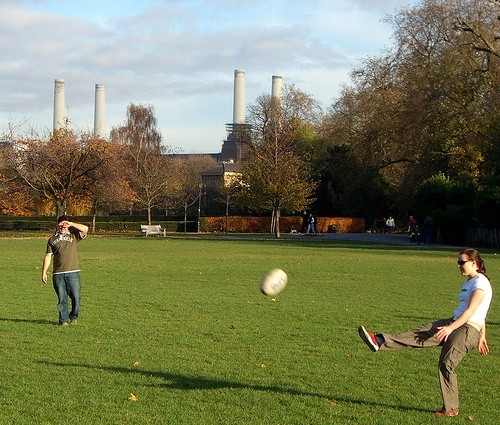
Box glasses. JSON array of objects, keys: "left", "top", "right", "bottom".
[{"left": 458, "top": 261, "right": 470, "bottom": 265}]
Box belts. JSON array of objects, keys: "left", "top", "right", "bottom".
[{"left": 451, "top": 315, "right": 481, "bottom": 333}]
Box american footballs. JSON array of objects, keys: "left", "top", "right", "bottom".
[{"left": 259, "top": 268, "right": 289, "bottom": 297}]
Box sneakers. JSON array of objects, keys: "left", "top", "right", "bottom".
[
  {"left": 358, "top": 325, "right": 380, "bottom": 352},
  {"left": 435, "top": 409, "right": 459, "bottom": 416}
]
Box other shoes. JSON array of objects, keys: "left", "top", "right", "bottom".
[
  {"left": 60, "top": 322, "right": 68, "bottom": 326},
  {"left": 70, "top": 320, "right": 78, "bottom": 325}
]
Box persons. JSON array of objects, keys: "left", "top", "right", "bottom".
[
  {"left": 299, "top": 210, "right": 316, "bottom": 236},
  {"left": 378, "top": 216, "right": 396, "bottom": 235},
  {"left": 41, "top": 215, "right": 89, "bottom": 327},
  {"left": 408, "top": 215, "right": 418, "bottom": 244},
  {"left": 421, "top": 214, "right": 434, "bottom": 245},
  {"left": 359, "top": 249, "right": 492, "bottom": 417}
]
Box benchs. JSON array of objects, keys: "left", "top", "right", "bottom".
[{"left": 138, "top": 224, "right": 167, "bottom": 237}]
